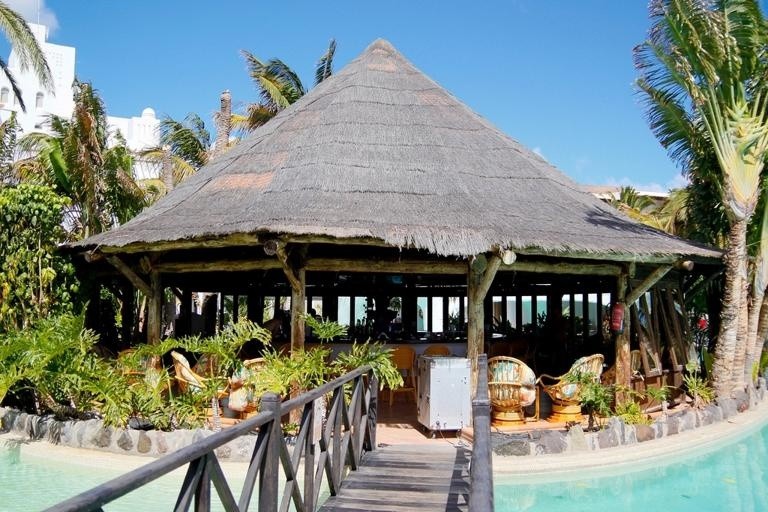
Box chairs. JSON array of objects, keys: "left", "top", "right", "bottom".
[
  {"left": 487, "top": 356, "right": 540, "bottom": 425},
  {"left": 423, "top": 344, "right": 451, "bottom": 356},
  {"left": 380, "top": 344, "right": 417, "bottom": 406},
  {"left": 540, "top": 353, "right": 605, "bottom": 424},
  {"left": 231, "top": 358, "right": 267, "bottom": 419},
  {"left": 118, "top": 350, "right": 167, "bottom": 417},
  {"left": 171, "top": 351, "right": 229, "bottom": 402},
  {"left": 603, "top": 350, "right": 642, "bottom": 383}
]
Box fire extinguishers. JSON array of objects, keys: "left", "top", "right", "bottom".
[{"left": 610, "top": 299, "right": 625, "bottom": 334}]
